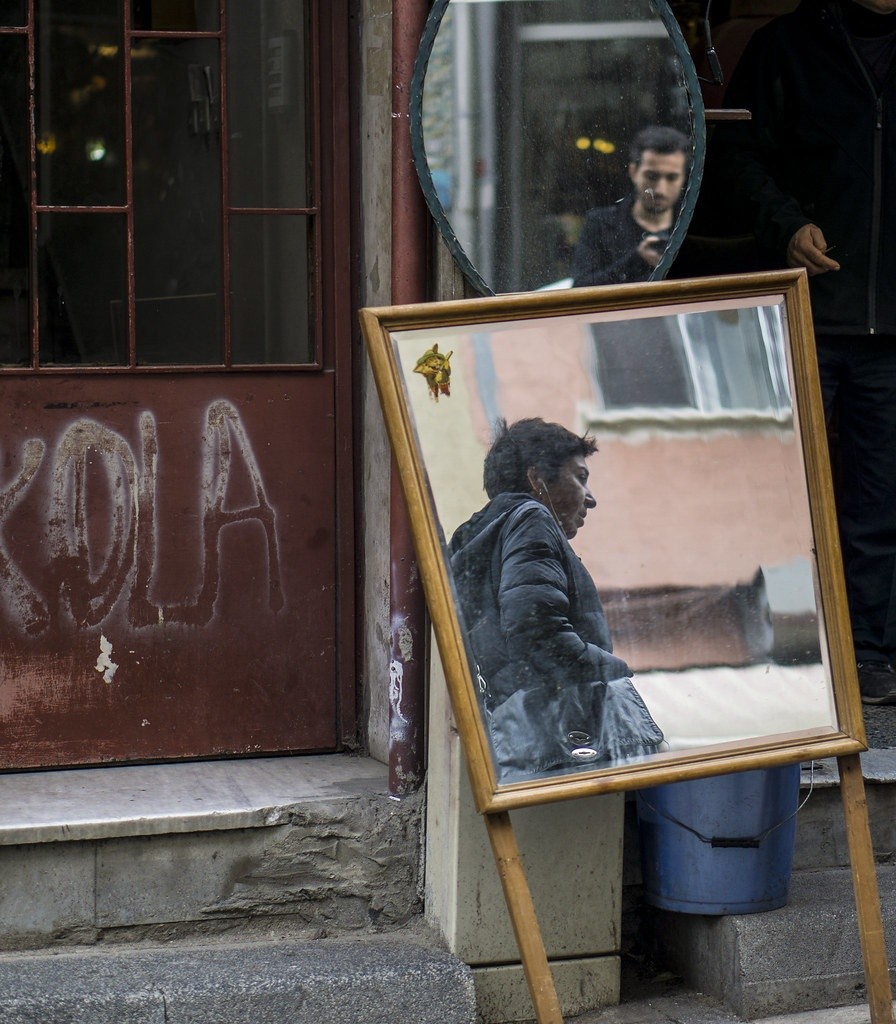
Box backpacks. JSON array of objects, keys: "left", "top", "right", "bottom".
[{"left": 484, "top": 680, "right": 671, "bottom": 783}]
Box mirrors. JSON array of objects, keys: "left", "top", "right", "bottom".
[
  {"left": 409, "top": 0, "right": 706, "bottom": 294},
  {"left": 358, "top": 267, "right": 871, "bottom": 813}
]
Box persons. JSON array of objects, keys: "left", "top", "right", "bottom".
[
  {"left": 708, "top": 0, "right": 896, "bottom": 707},
  {"left": 447, "top": 419, "right": 667, "bottom": 778},
  {"left": 570, "top": 125, "right": 691, "bottom": 288}
]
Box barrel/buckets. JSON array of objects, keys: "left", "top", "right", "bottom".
[{"left": 635, "top": 754, "right": 823, "bottom": 913}]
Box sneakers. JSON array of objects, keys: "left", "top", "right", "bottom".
[{"left": 857, "top": 660, "right": 896, "bottom": 705}]
